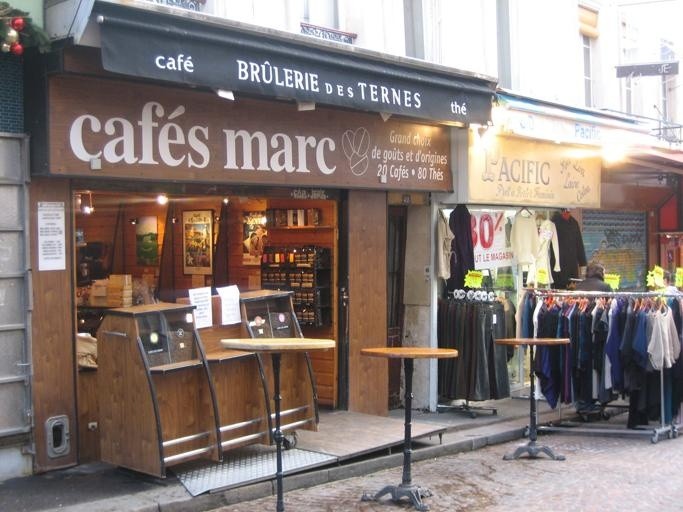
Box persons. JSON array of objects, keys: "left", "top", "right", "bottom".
[
  {"left": 574, "top": 263, "right": 613, "bottom": 292},
  {"left": 657, "top": 272, "right": 683, "bottom": 295}
]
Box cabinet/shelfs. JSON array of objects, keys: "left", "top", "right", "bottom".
[
  {"left": 261, "top": 199, "right": 338, "bottom": 409},
  {"left": 96, "top": 289, "right": 318, "bottom": 479}
]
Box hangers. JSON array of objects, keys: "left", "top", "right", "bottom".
[
  {"left": 515, "top": 207, "right": 532, "bottom": 215},
  {"left": 561, "top": 208, "right": 572, "bottom": 218},
  {"left": 566, "top": 282, "right": 577, "bottom": 288},
  {"left": 536, "top": 212, "right": 547, "bottom": 220},
  {"left": 438, "top": 290, "right": 672, "bottom": 312}
]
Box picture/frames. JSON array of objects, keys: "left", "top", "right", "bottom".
[{"left": 182, "top": 210, "right": 213, "bottom": 274}]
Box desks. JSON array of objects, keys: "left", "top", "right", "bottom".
[
  {"left": 495, "top": 337, "right": 571, "bottom": 460},
  {"left": 219, "top": 338, "right": 336, "bottom": 512},
  {"left": 360, "top": 347, "right": 459, "bottom": 512}
]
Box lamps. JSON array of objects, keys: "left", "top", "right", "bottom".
[
  {"left": 80, "top": 192, "right": 94, "bottom": 216},
  {"left": 168, "top": 216, "right": 176, "bottom": 225},
  {"left": 128, "top": 218, "right": 139, "bottom": 225}
]
